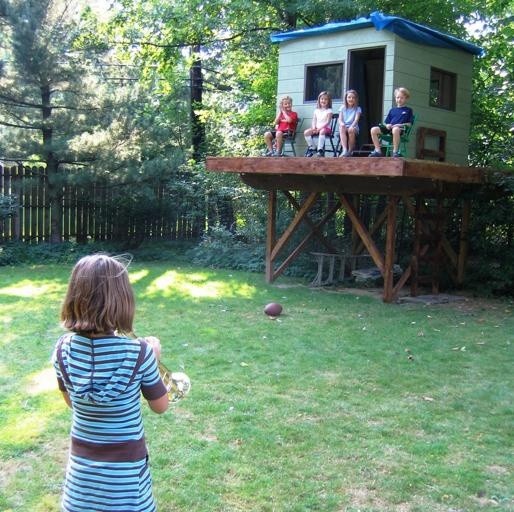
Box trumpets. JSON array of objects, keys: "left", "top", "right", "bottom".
[{"left": 119, "top": 322, "right": 191, "bottom": 405}]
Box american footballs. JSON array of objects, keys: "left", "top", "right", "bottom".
[{"left": 264, "top": 303, "right": 282, "bottom": 315}]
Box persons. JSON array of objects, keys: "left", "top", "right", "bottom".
[
  {"left": 336, "top": 88, "right": 362, "bottom": 157},
  {"left": 258, "top": 94, "right": 298, "bottom": 159},
  {"left": 366, "top": 87, "right": 414, "bottom": 159},
  {"left": 302, "top": 90, "right": 334, "bottom": 158},
  {"left": 49, "top": 250, "right": 173, "bottom": 512}
]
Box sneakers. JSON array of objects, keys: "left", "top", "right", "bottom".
[
  {"left": 264, "top": 151, "right": 282, "bottom": 156},
  {"left": 369, "top": 150, "right": 382, "bottom": 157},
  {"left": 307, "top": 147, "right": 325, "bottom": 157},
  {"left": 339, "top": 150, "right": 351, "bottom": 157},
  {"left": 393, "top": 152, "right": 398, "bottom": 156}
]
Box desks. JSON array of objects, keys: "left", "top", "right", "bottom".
[{"left": 307, "top": 250, "right": 373, "bottom": 289}]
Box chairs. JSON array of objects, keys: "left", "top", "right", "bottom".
[{"left": 264, "top": 112, "right": 421, "bottom": 159}]
